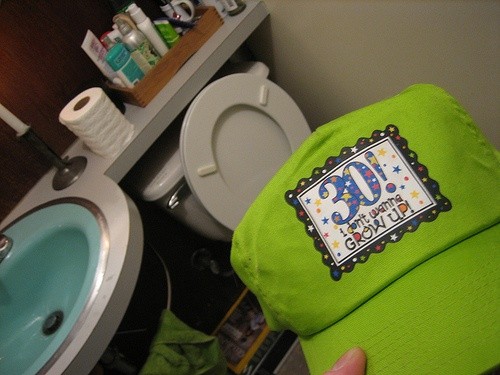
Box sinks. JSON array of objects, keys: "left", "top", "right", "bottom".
[{"left": 0, "top": 196, "right": 111, "bottom": 374}]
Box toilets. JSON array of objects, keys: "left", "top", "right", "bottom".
[{"left": 130, "top": 56, "right": 318, "bottom": 243}]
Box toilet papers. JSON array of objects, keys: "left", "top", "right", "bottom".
[{"left": 57, "top": 85, "right": 134, "bottom": 158}]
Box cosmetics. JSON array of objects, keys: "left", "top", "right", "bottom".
[
  {"left": 81, "top": 29, "right": 123, "bottom": 85},
  {"left": 105, "top": 42, "right": 143, "bottom": 88},
  {"left": 98, "top": 0, "right": 182, "bottom": 76}
]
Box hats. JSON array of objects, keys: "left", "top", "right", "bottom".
[{"left": 230, "top": 83, "right": 499, "bottom": 375}]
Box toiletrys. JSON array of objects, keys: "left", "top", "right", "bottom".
[{"left": 70, "top": 0, "right": 200, "bottom": 85}]
